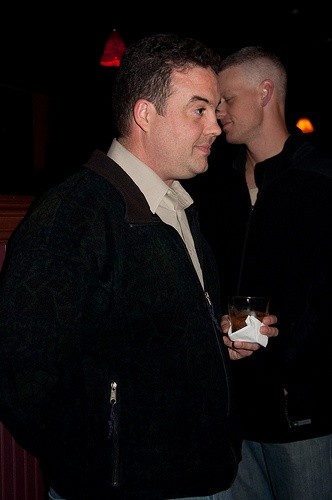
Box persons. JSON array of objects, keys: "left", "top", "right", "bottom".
[
  {"left": 1, "top": 33, "right": 279, "bottom": 500},
  {"left": 209, "top": 47, "right": 332, "bottom": 500}
]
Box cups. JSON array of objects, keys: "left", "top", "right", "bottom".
[{"left": 229, "top": 295, "right": 271, "bottom": 333}]
